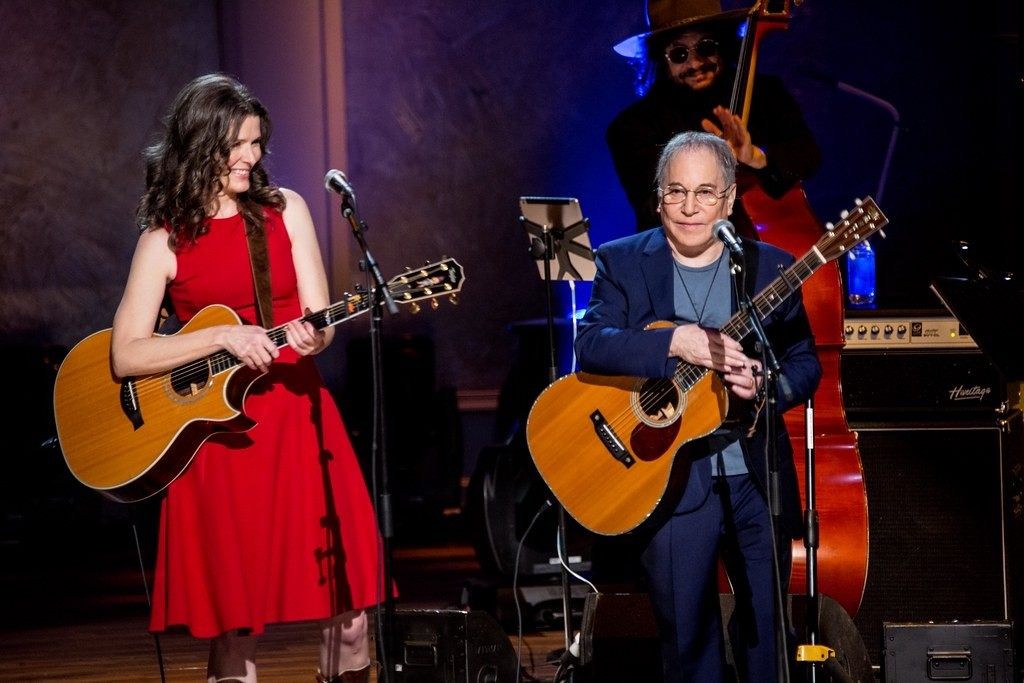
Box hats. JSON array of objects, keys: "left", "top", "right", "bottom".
[{"left": 645, "top": 0, "right": 751, "bottom": 43}]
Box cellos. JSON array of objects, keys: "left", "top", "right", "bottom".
[{"left": 712, "top": 0, "right": 873, "bottom": 634}]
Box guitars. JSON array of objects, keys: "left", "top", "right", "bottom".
[
  {"left": 523, "top": 192, "right": 893, "bottom": 547},
  {"left": 52, "top": 253, "right": 467, "bottom": 506}
]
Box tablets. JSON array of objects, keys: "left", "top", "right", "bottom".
[{"left": 520, "top": 195, "right": 596, "bottom": 281}]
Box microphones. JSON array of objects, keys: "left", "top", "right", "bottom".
[
  {"left": 324, "top": 168, "right": 356, "bottom": 198},
  {"left": 711, "top": 219, "right": 745, "bottom": 257}
]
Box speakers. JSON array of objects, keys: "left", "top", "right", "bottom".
[
  {"left": 578, "top": 592, "right": 877, "bottom": 682},
  {"left": 372, "top": 607, "right": 529, "bottom": 683},
  {"left": 846, "top": 418, "right": 1011, "bottom": 675}
]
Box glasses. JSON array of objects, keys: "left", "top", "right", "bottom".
[
  {"left": 663, "top": 185, "right": 730, "bottom": 206},
  {"left": 665, "top": 38, "right": 720, "bottom": 64}
]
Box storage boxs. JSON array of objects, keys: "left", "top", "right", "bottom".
[{"left": 883, "top": 620, "right": 1024, "bottom": 683}]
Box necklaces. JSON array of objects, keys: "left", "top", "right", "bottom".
[{"left": 674, "top": 245, "right": 726, "bottom": 325}]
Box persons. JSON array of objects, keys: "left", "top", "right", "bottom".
[
  {"left": 606, "top": 0, "right": 824, "bottom": 231},
  {"left": 111, "top": 74, "right": 398, "bottom": 683},
  {"left": 572, "top": 131, "right": 824, "bottom": 683}
]
